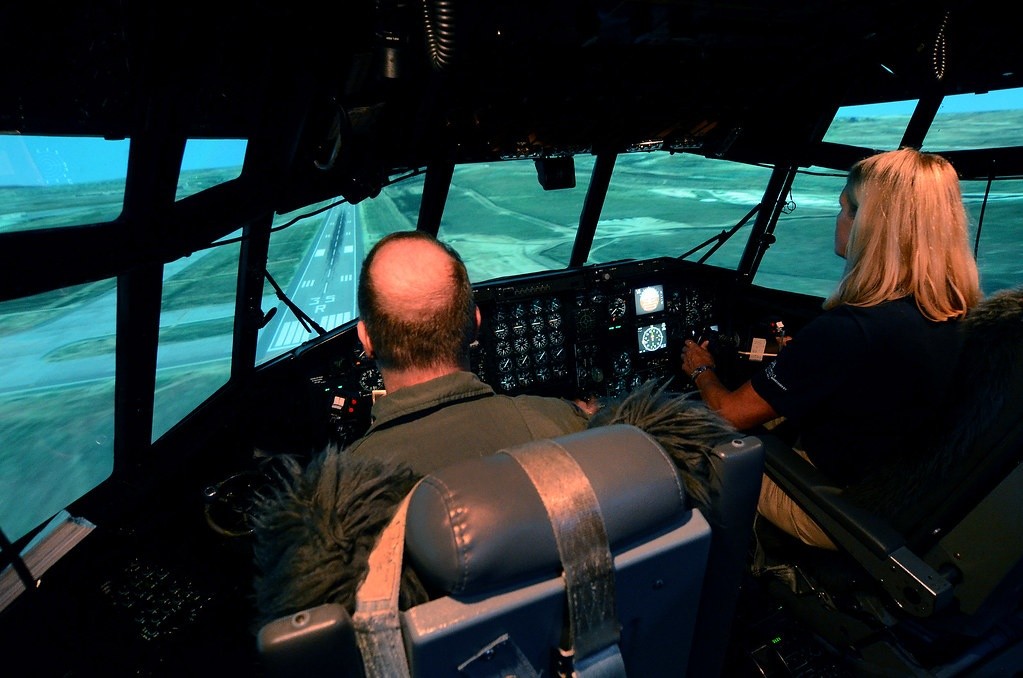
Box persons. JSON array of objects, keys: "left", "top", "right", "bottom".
[
  {"left": 240, "top": 232, "right": 591, "bottom": 639},
  {"left": 680, "top": 148, "right": 983, "bottom": 549}
]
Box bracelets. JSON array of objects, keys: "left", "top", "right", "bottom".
[{"left": 691, "top": 365, "right": 713, "bottom": 381}]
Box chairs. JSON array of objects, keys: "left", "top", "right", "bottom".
[
  {"left": 740, "top": 423, "right": 1023, "bottom": 678},
  {"left": 257, "top": 421, "right": 765, "bottom": 678}
]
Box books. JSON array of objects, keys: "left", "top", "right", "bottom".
[{"left": 0, "top": 509, "right": 97, "bottom": 615}]
